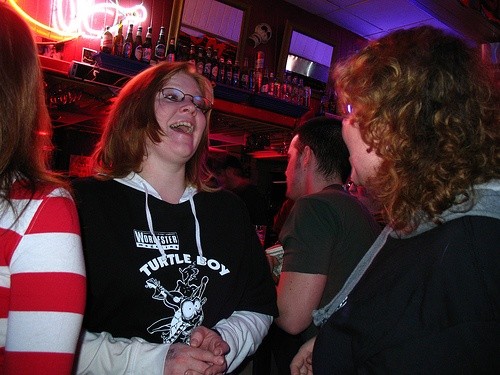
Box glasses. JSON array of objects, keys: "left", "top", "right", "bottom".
[{"left": 159, "top": 87, "right": 215, "bottom": 114}]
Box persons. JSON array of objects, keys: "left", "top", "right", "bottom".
[
  {"left": 71, "top": 61, "right": 280, "bottom": 375},
  {"left": 0, "top": 3, "right": 87, "bottom": 375},
  {"left": 213, "top": 117, "right": 382, "bottom": 375},
  {"left": 290, "top": 25, "right": 500, "bottom": 375}
]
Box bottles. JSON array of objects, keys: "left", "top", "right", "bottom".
[
  {"left": 233, "top": 55, "right": 241, "bottom": 88},
  {"left": 178, "top": 46, "right": 187, "bottom": 63},
  {"left": 113, "top": 19, "right": 124, "bottom": 57},
  {"left": 134, "top": 26, "right": 143, "bottom": 62},
  {"left": 218, "top": 53, "right": 226, "bottom": 84},
  {"left": 250, "top": 65, "right": 312, "bottom": 110},
  {"left": 166, "top": 37, "right": 177, "bottom": 62},
  {"left": 226, "top": 55, "right": 233, "bottom": 86},
  {"left": 196, "top": 46, "right": 204, "bottom": 75},
  {"left": 241, "top": 57, "right": 249, "bottom": 89},
  {"left": 124, "top": 24, "right": 134, "bottom": 59},
  {"left": 204, "top": 47, "right": 212, "bottom": 80},
  {"left": 188, "top": 45, "right": 196, "bottom": 72},
  {"left": 211, "top": 50, "right": 219, "bottom": 83},
  {"left": 142, "top": 26, "right": 153, "bottom": 64},
  {"left": 100, "top": 26, "right": 113, "bottom": 54},
  {"left": 153, "top": 26, "right": 166, "bottom": 64}
]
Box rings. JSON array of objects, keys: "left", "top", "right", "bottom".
[{"left": 184, "top": 369, "right": 190, "bottom": 375}]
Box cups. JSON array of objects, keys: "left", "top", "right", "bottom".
[{"left": 253, "top": 224, "right": 266, "bottom": 247}]
